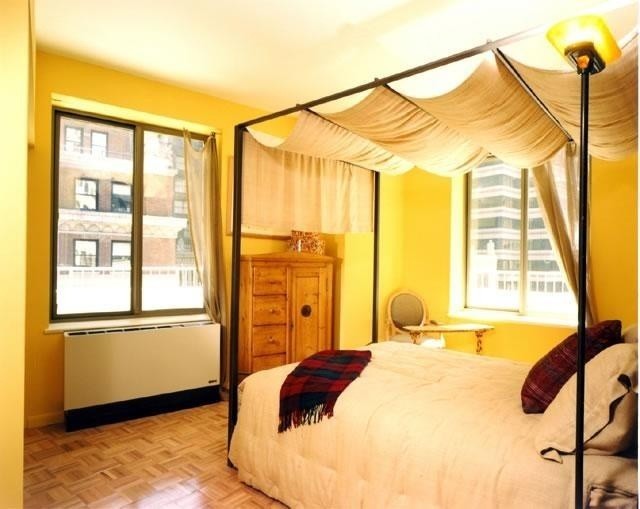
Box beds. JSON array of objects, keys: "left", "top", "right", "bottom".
[{"left": 226, "top": 0, "right": 638, "bottom": 509}]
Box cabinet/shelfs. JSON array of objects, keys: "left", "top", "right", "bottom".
[{"left": 238, "top": 256, "right": 334, "bottom": 384}]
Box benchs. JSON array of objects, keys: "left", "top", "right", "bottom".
[{"left": 402, "top": 323, "right": 495, "bottom": 356}]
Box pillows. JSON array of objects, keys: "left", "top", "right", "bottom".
[
  {"left": 521, "top": 319, "right": 622, "bottom": 415},
  {"left": 533, "top": 343, "right": 638, "bottom": 464},
  {"left": 621, "top": 325, "right": 638, "bottom": 343}
]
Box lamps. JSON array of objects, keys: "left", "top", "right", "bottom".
[{"left": 546, "top": 14, "right": 622, "bottom": 509}]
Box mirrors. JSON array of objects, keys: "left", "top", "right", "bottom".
[{"left": 386, "top": 291, "right": 426, "bottom": 334}]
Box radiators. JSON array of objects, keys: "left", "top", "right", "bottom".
[{"left": 64, "top": 321, "right": 221, "bottom": 433}]
